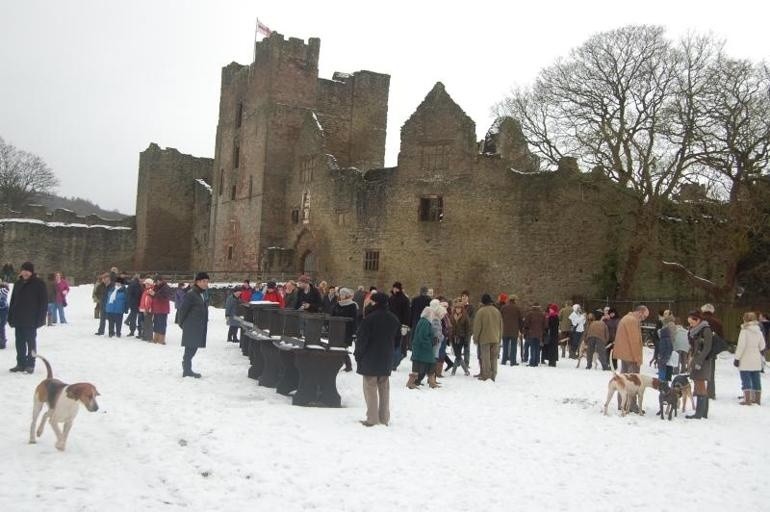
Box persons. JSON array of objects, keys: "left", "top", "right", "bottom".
[
  {"left": 225, "top": 275, "right": 622, "bottom": 389},
  {"left": 174, "top": 271, "right": 210, "bottom": 378},
  {"left": 612, "top": 303, "right": 725, "bottom": 419},
  {"left": 354, "top": 292, "right": 402, "bottom": 427},
  {"left": 93, "top": 267, "right": 172, "bottom": 345},
  {"left": 1, "top": 262, "right": 70, "bottom": 375},
  {"left": 176, "top": 282, "right": 210, "bottom": 322},
  {"left": 734, "top": 312, "right": 770, "bottom": 406}
]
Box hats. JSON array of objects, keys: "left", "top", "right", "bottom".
[
  {"left": 195, "top": 272, "right": 210, "bottom": 280},
  {"left": 98, "top": 268, "right": 164, "bottom": 286},
  {"left": 701, "top": 304, "right": 715, "bottom": 314},
  {"left": 608, "top": 309, "right": 616, "bottom": 314},
  {"left": 548, "top": 304, "right": 559, "bottom": 312},
  {"left": 663, "top": 310, "right": 675, "bottom": 322},
  {"left": 232, "top": 277, "right": 519, "bottom": 323},
  {"left": 20, "top": 262, "right": 36, "bottom": 273},
  {"left": 532, "top": 302, "right": 541, "bottom": 308}
]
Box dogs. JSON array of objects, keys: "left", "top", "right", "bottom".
[
  {"left": 670, "top": 373, "right": 696, "bottom": 414},
  {"left": 603, "top": 348, "right": 661, "bottom": 417},
  {"left": 28, "top": 352, "right": 101, "bottom": 451},
  {"left": 658, "top": 385, "right": 679, "bottom": 420}
]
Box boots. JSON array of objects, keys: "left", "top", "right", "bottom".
[
  {"left": 737, "top": 388, "right": 761, "bottom": 405},
  {"left": 685, "top": 395, "right": 710, "bottom": 419}
]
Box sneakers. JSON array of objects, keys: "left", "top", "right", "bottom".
[
  {"left": 93, "top": 313, "right": 166, "bottom": 344},
  {"left": 0, "top": 318, "right": 68, "bottom": 349},
  {"left": 9, "top": 365, "right": 35, "bottom": 374},
  {"left": 404, "top": 351, "right": 690, "bottom": 389},
  {"left": 360, "top": 419, "right": 389, "bottom": 427},
  {"left": 227, "top": 337, "right": 241, "bottom": 343},
  {"left": 183, "top": 371, "right": 202, "bottom": 378}
]
